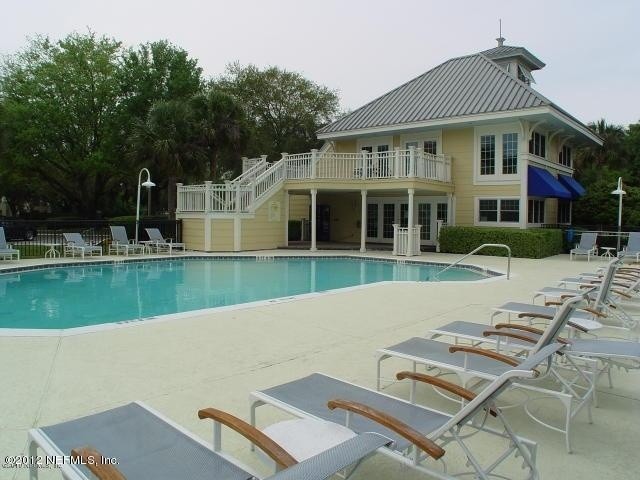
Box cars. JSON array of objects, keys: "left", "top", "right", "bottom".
[{"left": 0, "top": 218, "right": 38, "bottom": 243}]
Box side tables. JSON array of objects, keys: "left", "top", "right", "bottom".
[
  {"left": 254, "top": 417, "right": 358, "bottom": 480},
  {"left": 138, "top": 241, "right": 154, "bottom": 255},
  {"left": 41, "top": 244, "right": 63, "bottom": 260}
]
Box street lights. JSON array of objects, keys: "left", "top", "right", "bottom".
[
  {"left": 610, "top": 176, "right": 629, "bottom": 260},
  {"left": 135, "top": 166, "right": 157, "bottom": 254}
]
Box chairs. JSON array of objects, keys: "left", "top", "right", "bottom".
[
  {"left": 108, "top": 225, "right": 145, "bottom": 257},
  {"left": 26, "top": 399, "right": 395, "bottom": 480},
  {"left": 62, "top": 232, "right": 102, "bottom": 260},
  {"left": 143, "top": 227, "right": 186, "bottom": 254},
  {"left": 249, "top": 341, "right": 566, "bottom": 480},
  {"left": 0, "top": 226, "right": 20, "bottom": 264},
  {"left": 375, "top": 231, "right": 640, "bottom": 455}
]
